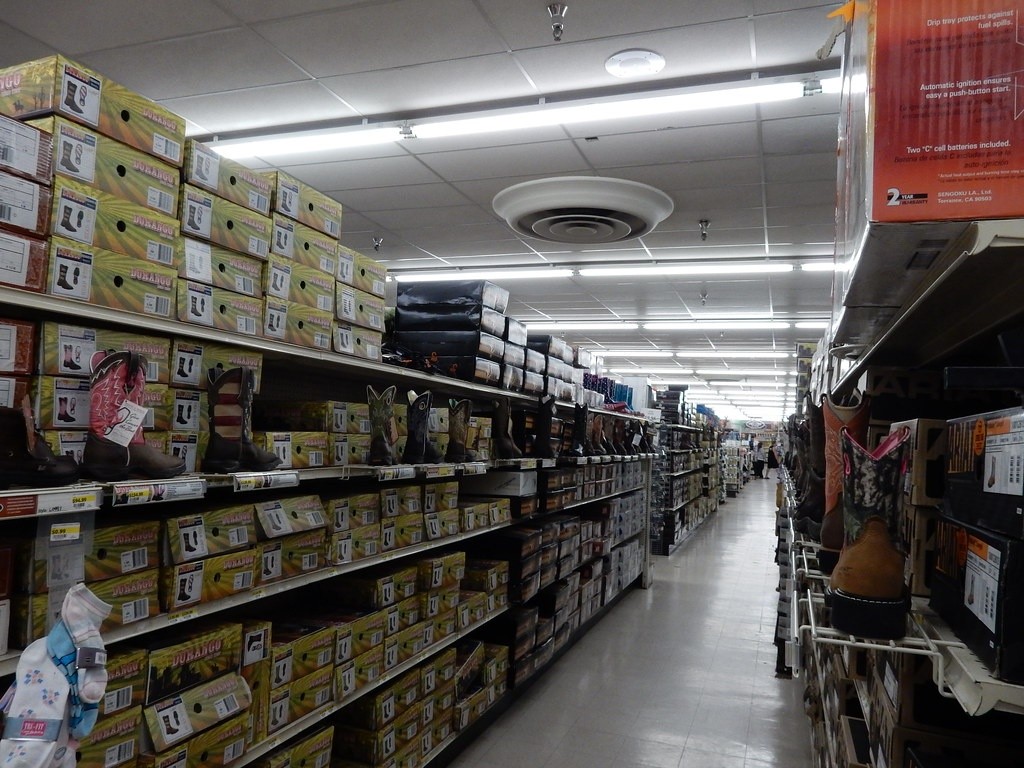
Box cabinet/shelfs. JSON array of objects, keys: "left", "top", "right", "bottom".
[
  {"left": 0, "top": 286, "right": 655, "bottom": 768},
  {"left": 720, "top": 425, "right": 783, "bottom": 498},
  {"left": 655, "top": 423, "right": 728, "bottom": 556},
  {"left": 782, "top": 218, "right": 1024, "bottom": 768}
]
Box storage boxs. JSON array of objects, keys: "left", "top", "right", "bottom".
[
  {"left": 871, "top": 698, "right": 1024, "bottom": 768},
  {"left": 794, "top": 1, "right": 1024, "bottom": 418},
  {"left": 460, "top": 462, "right": 645, "bottom": 517},
  {"left": 867, "top": 648, "right": 878, "bottom": 700},
  {"left": 929, "top": 512, "right": 1024, "bottom": 687},
  {"left": 839, "top": 638, "right": 867, "bottom": 680},
  {"left": 866, "top": 426, "right": 888, "bottom": 450},
  {"left": 663, "top": 427, "right": 721, "bottom": 544},
  {"left": 381, "top": 280, "right": 634, "bottom": 411},
  {"left": 945, "top": 405, "right": 1024, "bottom": 540},
  {"left": 876, "top": 643, "right": 1024, "bottom": 751},
  {"left": 836, "top": 714, "right": 871, "bottom": 768},
  {"left": 889, "top": 419, "right": 949, "bottom": 506},
  {"left": 896, "top": 504, "right": 944, "bottom": 597},
  {"left": 826, "top": 657, "right": 865, "bottom": 731},
  {"left": 622, "top": 376, "right": 708, "bottom": 428},
  {"left": 512, "top": 538, "right": 644, "bottom": 686},
  {"left": 0, "top": 55, "right": 387, "bottom": 363},
  {"left": 512, "top": 490, "right": 645, "bottom": 604},
  {"left": 0, "top": 482, "right": 511, "bottom": 656},
  {"left": 1, "top": 319, "right": 493, "bottom": 469},
  {"left": 833, "top": 653, "right": 866, "bottom": 734},
  {"left": 513, "top": 412, "right": 575, "bottom": 454},
  {"left": 867, "top": 367, "right": 946, "bottom": 425},
  {"left": 75, "top": 551, "right": 509, "bottom": 768},
  {"left": 816, "top": 583, "right": 832, "bottom": 627}
]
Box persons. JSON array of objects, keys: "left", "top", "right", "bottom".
[
  {"left": 749, "top": 436, "right": 765, "bottom": 479},
  {"left": 764, "top": 439, "right": 782, "bottom": 480}
]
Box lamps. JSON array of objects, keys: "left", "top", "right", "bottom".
[{"left": 210, "top": 77, "right": 843, "bottom": 159}]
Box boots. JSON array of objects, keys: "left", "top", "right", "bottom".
[
  {"left": 818, "top": 388, "right": 872, "bottom": 572},
  {"left": 524, "top": 394, "right": 556, "bottom": 459},
  {"left": 680, "top": 432, "right": 697, "bottom": 450},
  {"left": 489, "top": 397, "right": 523, "bottom": 460},
  {"left": 78, "top": 347, "right": 188, "bottom": 482},
  {"left": 784, "top": 387, "right": 826, "bottom": 542},
  {"left": 709, "top": 425, "right": 716, "bottom": 441},
  {"left": 823, "top": 423, "right": 914, "bottom": 641},
  {"left": 716, "top": 432, "right": 723, "bottom": 448},
  {"left": 559, "top": 401, "right": 656, "bottom": 457},
  {"left": 400, "top": 389, "right": 445, "bottom": 464},
  {"left": 444, "top": 398, "right": 488, "bottom": 464},
  {"left": 200, "top": 361, "right": 284, "bottom": 474},
  {"left": 366, "top": 385, "right": 402, "bottom": 467},
  {"left": 702, "top": 422, "right": 710, "bottom": 441}
]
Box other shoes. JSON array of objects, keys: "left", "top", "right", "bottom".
[
  {"left": 759, "top": 475, "right": 763, "bottom": 479},
  {"left": 776, "top": 476, "right": 781, "bottom": 479},
  {"left": 764, "top": 477, "right": 770, "bottom": 479}
]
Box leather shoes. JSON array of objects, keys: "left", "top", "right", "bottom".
[{"left": 0, "top": 405, "right": 80, "bottom": 491}]
